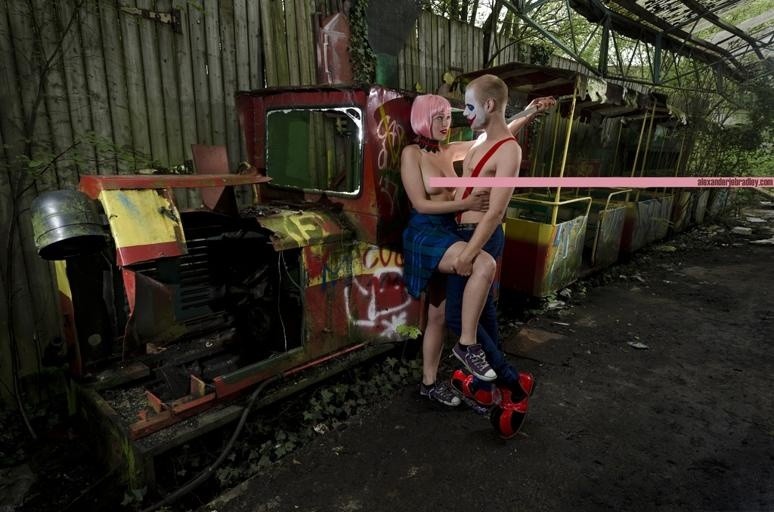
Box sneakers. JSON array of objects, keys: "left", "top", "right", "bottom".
[
  {"left": 451, "top": 341, "right": 497, "bottom": 382},
  {"left": 489, "top": 370, "right": 537, "bottom": 440},
  {"left": 419, "top": 380, "right": 461, "bottom": 407},
  {"left": 450, "top": 367, "right": 496, "bottom": 408}
]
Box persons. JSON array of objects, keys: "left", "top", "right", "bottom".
[
  {"left": 445, "top": 72, "right": 537, "bottom": 440},
  {"left": 400, "top": 94, "right": 556, "bottom": 406}
]
